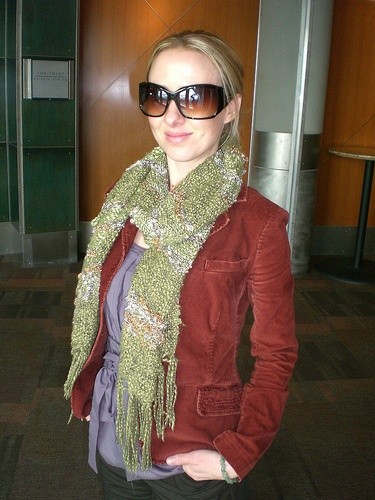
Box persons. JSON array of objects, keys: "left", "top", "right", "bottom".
[{"left": 58, "top": 29, "right": 298, "bottom": 499}]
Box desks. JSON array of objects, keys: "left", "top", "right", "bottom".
[{"left": 313, "top": 144, "right": 375, "bottom": 285}]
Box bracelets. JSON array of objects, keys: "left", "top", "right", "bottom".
[{"left": 220, "top": 453, "right": 240, "bottom": 485}]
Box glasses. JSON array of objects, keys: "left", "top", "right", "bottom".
[{"left": 136, "top": 80, "right": 234, "bottom": 119}]
